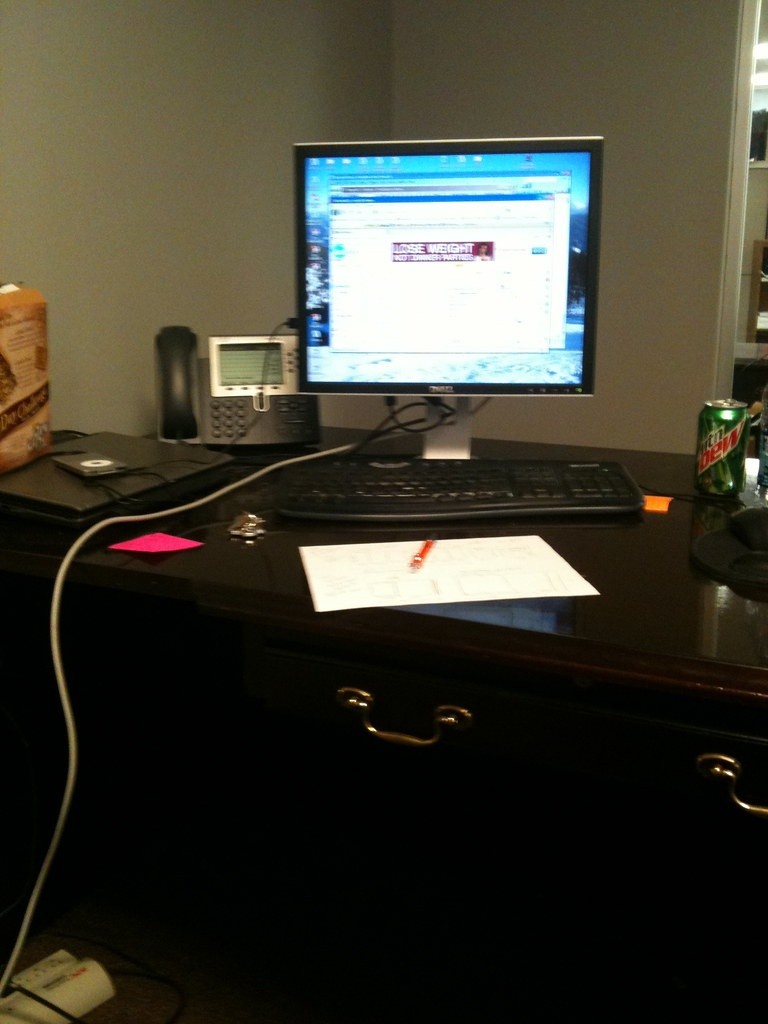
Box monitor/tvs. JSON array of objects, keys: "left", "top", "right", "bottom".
[{"left": 293, "top": 136, "right": 605, "bottom": 460}]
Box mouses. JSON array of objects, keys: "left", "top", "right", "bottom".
[{"left": 727, "top": 507, "right": 768, "bottom": 551}]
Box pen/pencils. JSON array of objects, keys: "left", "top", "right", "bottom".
[{"left": 409, "top": 539, "right": 433, "bottom": 569}]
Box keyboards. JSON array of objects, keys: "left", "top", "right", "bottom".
[{"left": 270, "top": 459, "right": 644, "bottom": 523}]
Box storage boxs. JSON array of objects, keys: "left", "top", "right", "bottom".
[{"left": 0, "top": 280, "right": 51, "bottom": 475}]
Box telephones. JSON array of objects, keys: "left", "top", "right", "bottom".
[{"left": 155, "top": 326, "right": 320, "bottom": 445}]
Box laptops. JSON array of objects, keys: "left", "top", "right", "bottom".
[{"left": 0, "top": 431, "right": 235, "bottom": 529}]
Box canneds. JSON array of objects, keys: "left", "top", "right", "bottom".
[{"left": 694, "top": 398, "right": 751, "bottom": 496}]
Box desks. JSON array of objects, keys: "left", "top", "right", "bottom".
[{"left": 0, "top": 427, "right": 768, "bottom": 957}]
